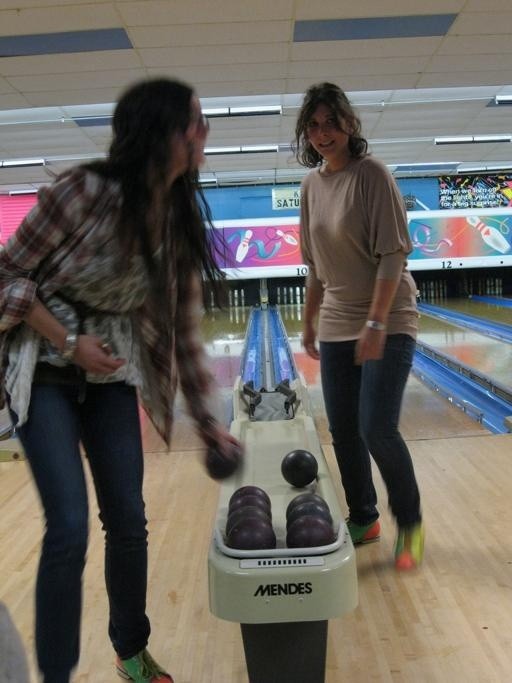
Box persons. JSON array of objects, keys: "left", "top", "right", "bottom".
[
  {"left": 290, "top": 77, "right": 430, "bottom": 573},
  {"left": 2, "top": 69, "right": 246, "bottom": 683}
]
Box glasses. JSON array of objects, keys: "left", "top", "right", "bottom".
[{"left": 189, "top": 114, "right": 211, "bottom": 146}]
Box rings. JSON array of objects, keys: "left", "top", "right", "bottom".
[{"left": 102, "top": 342, "right": 110, "bottom": 348}]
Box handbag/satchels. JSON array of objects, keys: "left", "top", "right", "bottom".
[{"left": 0, "top": 321, "right": 24, "bottom": 410}]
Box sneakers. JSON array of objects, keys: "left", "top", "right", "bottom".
[
  {"left": 115, "top": 649, "right": 173, "bottom": 683},
  {"left": 395, "top": 522, "right": 423, "bottom": 570},
  {"left": 346, "top": 518, "right": 381, "bottom": 545}
]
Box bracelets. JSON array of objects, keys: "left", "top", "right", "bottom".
[
  {"left": 365, "top": 318, "right": 386, "bottom": 331},
  {"left": 60, "top": 331, "right": 80, "bottom": 362}
]
